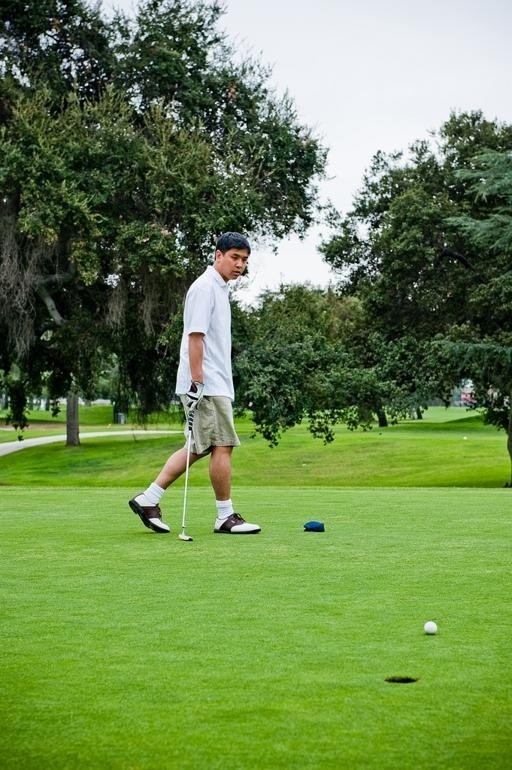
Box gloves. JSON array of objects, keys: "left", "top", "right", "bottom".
[{"left": 186, "top": 381, "right": 203, "bottom": 411}]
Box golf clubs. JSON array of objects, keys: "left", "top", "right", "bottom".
[{"left": 178, "top": 410, "right": 193, "bottom": 542}]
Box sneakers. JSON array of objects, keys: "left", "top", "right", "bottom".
[
  {"left": 214, "top": 514, "right": 261, "bottom": 535},
  {"left": 129, "top": 494, "right": 171, "bottom": 535}
]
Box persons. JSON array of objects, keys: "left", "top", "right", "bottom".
[{"left": 128, "top": 231, "right": 262, "bottom": 534}]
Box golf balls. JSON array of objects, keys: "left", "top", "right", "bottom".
[{"left": 424, "top": 621, "right": 437, "bottom": 634}]
